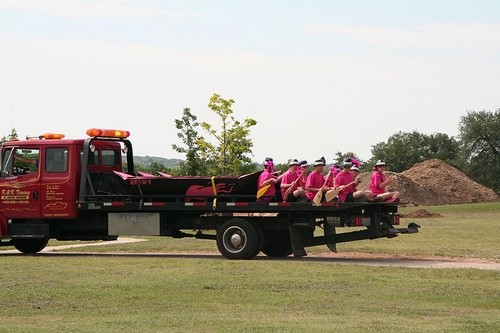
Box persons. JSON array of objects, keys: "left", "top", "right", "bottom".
[
  {"left": 369, "top": 160, "right": 399, "bottom": 202},
  {"left": 258, "top": 157, "right": 281, "bottom": 196},
  {"left": 350, "top": 164, "right": 361, "bottom": 175},
  {"left": 305, "top": 160, "right": 340, "bottom": 203},
  {"left": 335, "top": 157, "right": 373, "bottom": 201},
  {"left": 14, "top": 152, "right": 39, "bottom": 169},
  {"left": 280, "top": 159, "right": 309, "bottom": 202}
]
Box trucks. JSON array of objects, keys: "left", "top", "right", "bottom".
[{"left": 0, "top": 128, "right": 422, "bottom": 260}]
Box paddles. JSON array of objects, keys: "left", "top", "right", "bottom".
[
  {"left": 283, "top": 170, "right": 306, "bottom": 198},
  {"left": 325, "top": 179, "right": 356, "bottom": 202},
  {"left": 313, "top": 170, "right": 332, "bottom": 205},
  {"left": 257, "top": 169, "right": 289, "bottom": 198}
]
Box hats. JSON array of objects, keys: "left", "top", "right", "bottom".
[
  {"left": 263, "top": 158, "right": 274, "bottom": 166},
  {"left": 289, "top": 159, "right": 300, "bottom": 166},
  {"left": 336, "top": 165, "right": 341, "bottom": 171},
  {"left": 342, "top": 158, "right": 352, "bottom": 167},
  {"left": 313, "top": 160, "right": 326, "bottom": 167},
  {"left": 350, "top": 165, "right": 360, "bottom": 172},
  {"left": 299, "top": 161, "right": 310, "bottom": 167},
  {"left": 374, "top": 160, "right": 387, "bottom": 168}
]
